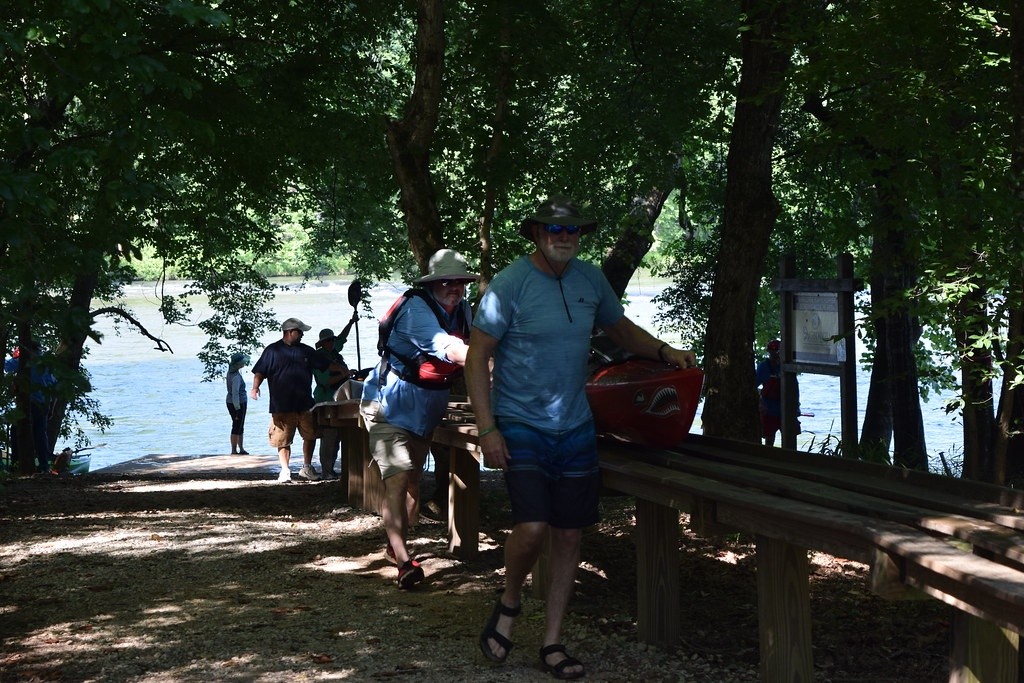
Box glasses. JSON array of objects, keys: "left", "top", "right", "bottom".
[
  {"left": 544, "top": 223, "right": 580, "bottom": 235},
  {"left": 443, "top": 279, "right": 465, "bottom": 287}
]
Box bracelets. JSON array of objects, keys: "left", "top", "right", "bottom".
[
  {"left": 658, "top": 343, "right": 669, "bottom": 362},
  {"left": 477, "top": 425, "right": 497, "bottom": 437}
]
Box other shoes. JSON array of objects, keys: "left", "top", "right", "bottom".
[
  {"left": 304, "top": 465, "right": 321, "bottom": 480},
  {"left": 240, "top": 451, "right": 249, "bottom": 455},
  {"left": 398, "top": 557, "right": 424, "bottom": 589},
  {"left": 387, "top": 544, "right": 399, "bottom": 564},
  {"left": 320, "top": 470, "right": 341, "bottom": 479},
  {"left": 277, "top": 470, "right": 292, "bottom": 484},
  {"left": 232, "top": 452, "right": 239, "bottom": 455}
]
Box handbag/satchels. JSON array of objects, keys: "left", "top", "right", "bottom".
[{"left": 351, "top": 368, "right": 374, "bottom": 381}]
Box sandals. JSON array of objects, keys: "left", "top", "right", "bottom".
[
  {"left": 479, "top": 595, "right": 521, "bottom": 663},
  {"left": 540, "top": 644, "right": 585, "bottom": 679}
]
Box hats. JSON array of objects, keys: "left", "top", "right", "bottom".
[
  {"left": 769, "top": 341, "right": 782, "bottom": 351},
  {"left": 229, "top": 353, "right": 250, "bottom": 373},
  {"left": 315, "top": 329, "right": 336, "bottom": 349},
  {"left": 283, "top": 319, "right": 311, "bottom": 331},
  {"left": 412, "top": 249, "right": 477, "bottom": 282},
  {"left": 520, "top": 195, "right": 598, "bottom": 242}
]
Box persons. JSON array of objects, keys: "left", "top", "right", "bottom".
[
  {"left": 251, "top": 318, "right": 322, "bottom": 483},
  {"left": 465, "top": 201, "right": 697, "bottom": 682},
  {"left": 226, "top": 353, "right": 250, "bottom": 455},
  {"left": 5, "top": 344, "right": 58, "bottom": 475},
  {"left": 360, "top": 249, "right": 493, "bottom": 589},
  {"left": 313, "top": 310, "right": 360, "bottom": 477},
  {"left": 755, "top": 341, "right": 799, "bottom": 447}
]
